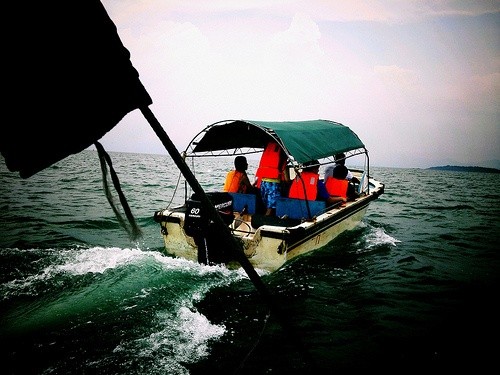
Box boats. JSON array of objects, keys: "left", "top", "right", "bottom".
[{"left": 154, "top": 119, "right": 385, "bottom": 273}]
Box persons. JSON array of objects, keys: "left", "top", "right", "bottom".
[
  {"left": 256, "top": 142, "right": 361, "bottom": 220},
  {"left": 225, "top": 156, "right": 252, "bottom": 193}
]
